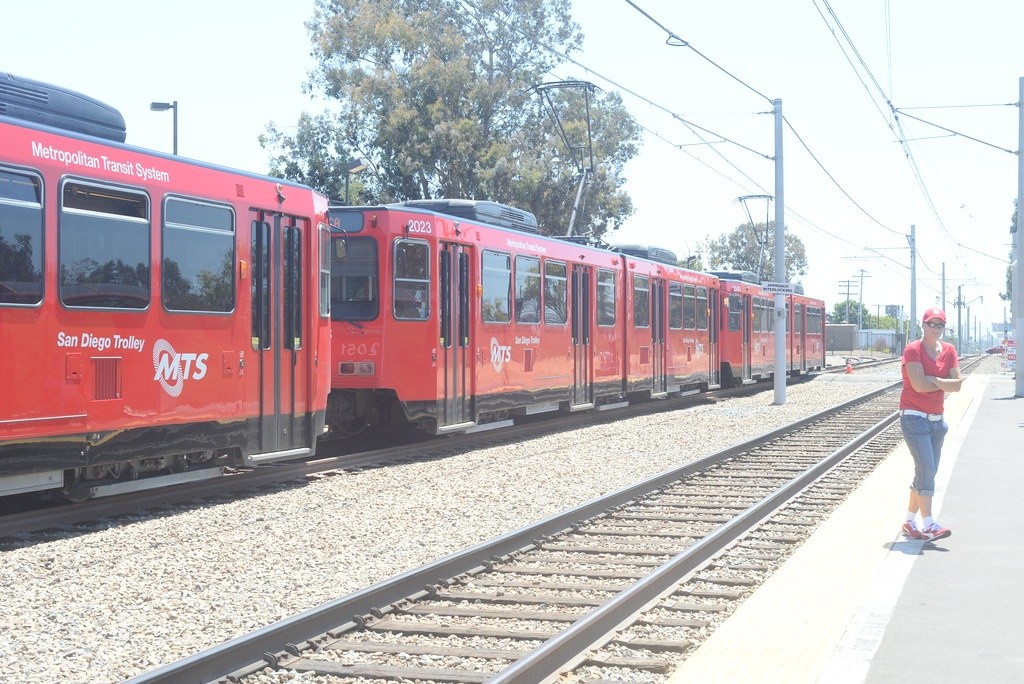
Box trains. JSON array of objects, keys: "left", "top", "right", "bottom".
[{"left": 0, "top": 79, "right": 826, "bottom": 504}]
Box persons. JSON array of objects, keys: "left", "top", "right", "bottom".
[{"left": 900, "top": 307, "right": 961, "bottom": 542}]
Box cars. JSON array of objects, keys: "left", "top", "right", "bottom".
[{"left": 986, "top": 345, "right": 1007, "bottom": 355}]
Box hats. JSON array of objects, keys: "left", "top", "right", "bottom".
[{"left": 923, "top": 307, "right": 946, "bottom": 324}]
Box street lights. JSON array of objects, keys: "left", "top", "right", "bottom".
[
  {"left": 344, "top": 159, "right": 364, "bottom": 206},
  {"left": 149, "top": 98, "right": 179, "bottom": 157}
]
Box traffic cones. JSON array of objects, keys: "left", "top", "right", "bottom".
[{"left": 844, "top": 360, "right": 853, "bottom": 374}]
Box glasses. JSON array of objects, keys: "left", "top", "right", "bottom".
[{"left": 924, "top": 322, "right": 945, "bottom": 328}]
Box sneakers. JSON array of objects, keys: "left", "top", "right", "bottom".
[
  {"left": 922, "top": 523, "right": 951, "bottom": 542},
  {"left": 901, "top": 522, "right": 923, "bottom": 540}
]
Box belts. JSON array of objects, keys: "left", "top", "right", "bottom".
[{"left": 900, "top": 409, "right": 944, "bottom": 422}]
